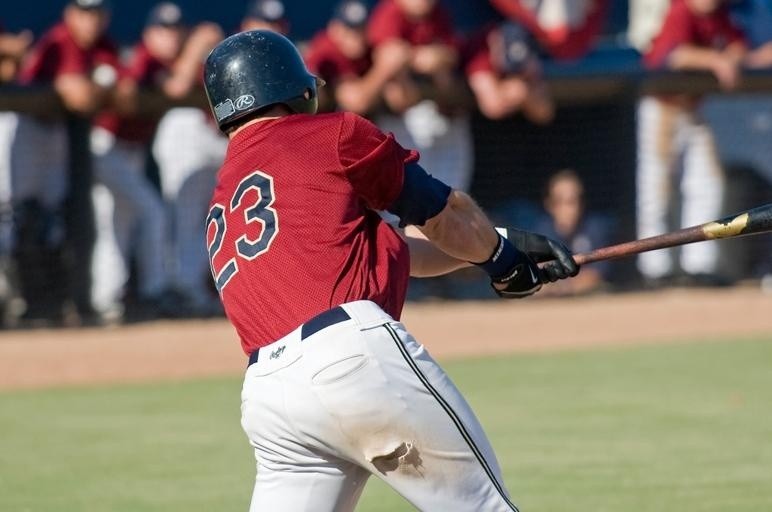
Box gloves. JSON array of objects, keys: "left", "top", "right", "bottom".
[
  {"left": 470, "top": 235, "right": 549, "bottom": 304},
  {"left": 502, "top": 223, "right": 580, "bottom": 284}
]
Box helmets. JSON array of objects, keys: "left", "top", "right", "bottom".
[{"left": 198, "top": 26, "right": 328, "bottom": 130}]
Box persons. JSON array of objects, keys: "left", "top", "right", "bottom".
[
  {"left": 634, "top": 1, "right": 772, "bottom": 284},
  {"left": 304, "top": 2, "right": 601, "bottom": 235},
  {"left": 200, "top": 30, "right": 579, "bottom": 509},
  {"left": 10, "top": 0, "right": 290, "bottom": 325}
]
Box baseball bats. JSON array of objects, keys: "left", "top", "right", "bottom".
[{"left": 494, "top": 204, "right": 771, "bottom": 290}]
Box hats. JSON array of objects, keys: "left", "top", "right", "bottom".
[{"left": 492, "top": 21, "right": 534, "bottom": 73}]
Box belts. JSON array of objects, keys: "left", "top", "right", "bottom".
[{"left": 247, "top": 305, "right": 352, "bottom": 368}]
